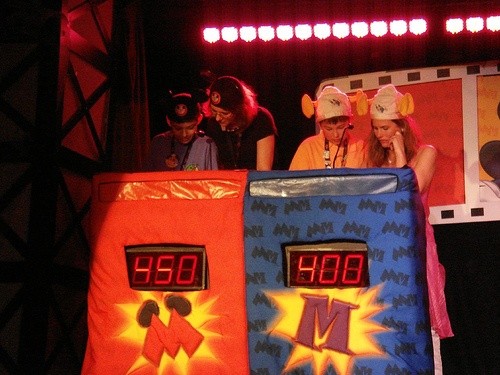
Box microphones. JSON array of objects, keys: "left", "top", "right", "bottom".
[
  {"left": 333, "top": 124, "right": 354, "bottom": 168},
  {"left": 206, "top": 139, "right": 212, "bottom": 170}
]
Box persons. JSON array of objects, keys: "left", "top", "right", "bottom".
[
  {"left": 358, "top": 84, "right": 454, "bottom": 375},
  {"left": 196, "top": 75, "right": 277, "bottom": 172},
  {"left": 290, "top": 87, "right": 376, "bottom": 171},
  {"left": 146, "top": 93, "right": 219, "bottom": 172}
]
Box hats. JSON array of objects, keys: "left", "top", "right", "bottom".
[
  {"left": 209, "top": 76, "right": 246, "bottom": 109},
  {"left": 166, "top": 93, "right": 200, "bottom": 123},
  {"left": 357, "top": 84, "right": 415, "bottom": 121},
  {"left": 301, "top": 86, "right": 363, "bottom": 124}
]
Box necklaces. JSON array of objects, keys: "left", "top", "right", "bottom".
[
  {"left": 324, "top": 135, "right": 348, "bottom": 168},
  {"left": 385, "top": 155, "right": 396, "bottom": 165},
  {"left": 224, "top": 124, "right": 244, "bottom": 170},
  {"left": 170, "top": 135, "right": 196, "bottom": 172}
]
